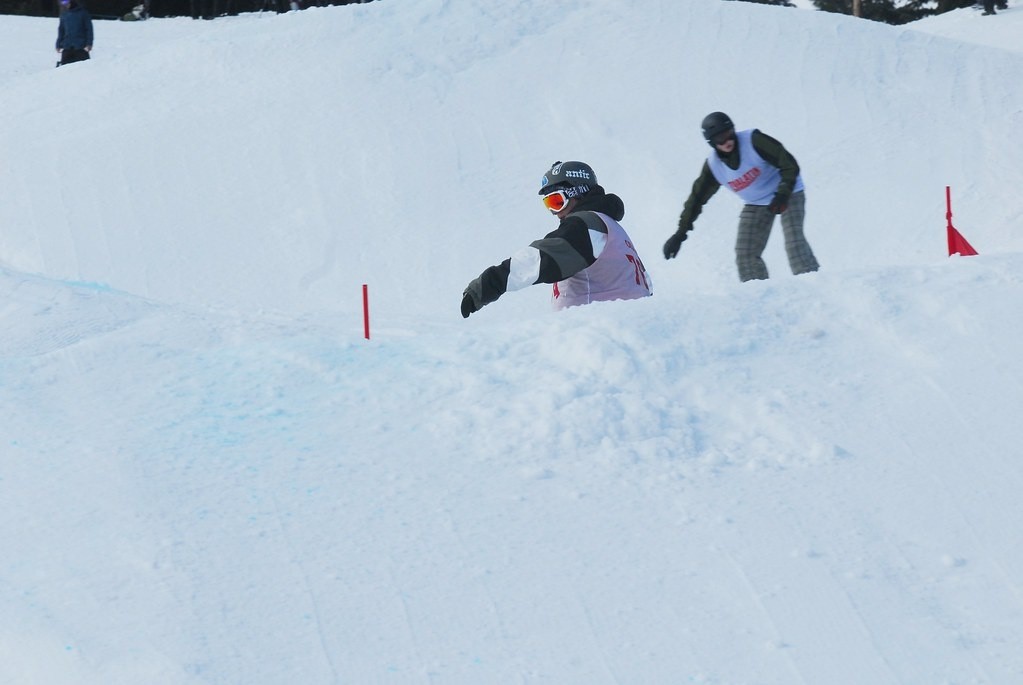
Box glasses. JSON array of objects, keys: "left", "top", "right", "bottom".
[
  {"left": 543, "top": 190, "right": 569, "bottom": 215},
  {"left": 710, "top": 127, "right": 734, "bottom": 145}
]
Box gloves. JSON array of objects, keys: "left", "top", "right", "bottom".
[
  {"left": 663, "top": 230, "right": 687, "bottom": 260},
  {"left": 461, "top": 294, "right": 477, "bottom": 318},
  {"left": 768, "top": 195, "right": 787, "bottom": 214}
]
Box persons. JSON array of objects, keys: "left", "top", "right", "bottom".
[
  {"left": 664, "top": 112, "right": 819, "bottom": 282},
  {"left": 460, "top": 161, "right": 653, "bottom": 318},
  {"left": 55, "top": 0, "right": 93, "bottom": 64}
]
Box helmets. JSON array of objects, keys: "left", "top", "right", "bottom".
[
  {"left": 538, "top": 161, "right": 597, "bottom": 198},
  {"left": 702, "top": 112, "right": 734, "bottom": 139}
]
